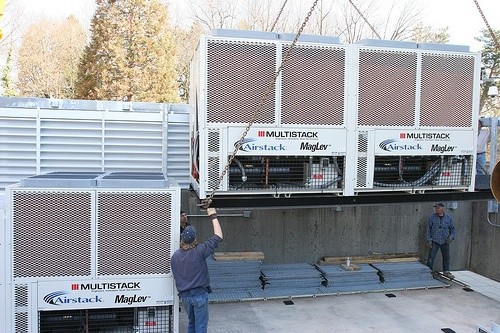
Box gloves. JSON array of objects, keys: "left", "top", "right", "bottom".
[
  {"left": 426, "top": 242, "right": 432, "bottom": 249},
  {"left": 207, "top": 208, "right": 217, "bottom": 220},
  {"left": 446, "top": 238, "right": 452, "bottom": 244}
]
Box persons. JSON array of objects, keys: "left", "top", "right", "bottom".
[
  {"left": 171, "top": 208, "right": 224, "bottom": 333},
  {"left": 425, "top": 202, "right": 456, "bottom": 279},
  {"left": 180, "top": 210, "right": 191, "bottom": 240}
]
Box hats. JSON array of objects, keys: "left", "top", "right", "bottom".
[
  {"left": 181, "top": 226, "right": 196, "bottom": 244},
  {"left": 433, "top": 203, "right": 444, "bottom": 207}
]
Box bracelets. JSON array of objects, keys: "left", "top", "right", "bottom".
[{"left": 210, "top": 213, "right": 217, "bottom": 221}]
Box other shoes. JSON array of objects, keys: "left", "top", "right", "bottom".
[{"left": 443, "top": 272, "right": 455, "bottom": 279}]
